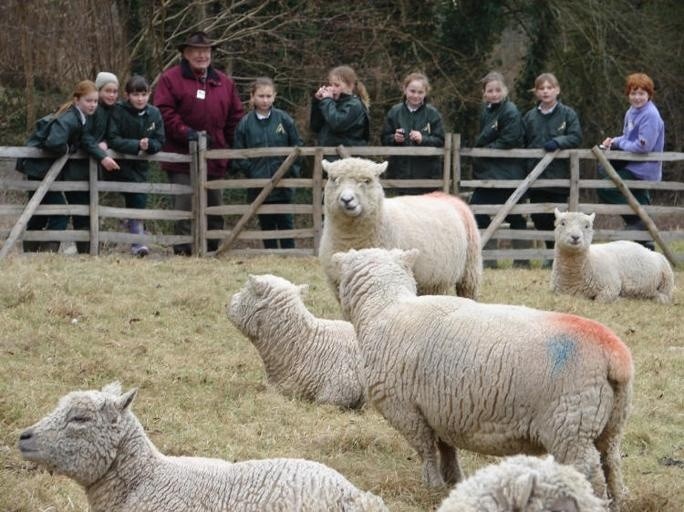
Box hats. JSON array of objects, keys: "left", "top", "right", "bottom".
[
  {"left": 95, "top": 71, "right": 120, "bottom": 90},
  {"left": 175, "top": 30, "right": 230, "bottom": 54}
]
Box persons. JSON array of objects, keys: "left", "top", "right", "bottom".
[
  {"left": 63, "top": 72, "right": 123, "bottom": 253},
  {"left": 378, "top": 71, "right": 446, "bottom": 195},
  {"left": 308, "top": 65, "right": 372, "bottom": 176},
  {"left": 15, "top": 79, "right": 100, "bottom": 253},
  {"left": 596, "top": 72, "right": 665, "bottom": 251},
  {"left": 467, "top": 69, "right": 531, "bottom": 268},
  {"left": 523, "top": 72, "right": 584, "bottom": 269},
  {"left": 152, "top": 30, "right": 245, "bottom": 257},
  {"left": 106, "top": 74, "right": 168, "bottom": 256},
  {"left": 233, "top": 76, "right": 305, "bottom": 248}
]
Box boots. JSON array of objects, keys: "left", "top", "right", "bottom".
[
  {"left": 511, "top": 239, "right": 533, "bottom": 270},
  {"left": 481, "top": 238, "right": 503, "bottom": 269},
  {"left": 128, "top": 219, "right": 150, "bottom": 257},
  {"left": 541, "top": 259, "right": 564, "bottom": 269},
  {"left": 625, "top": 220, "right": 658, "bottom": 249}
]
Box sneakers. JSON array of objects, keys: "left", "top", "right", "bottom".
[{"left": 207, "top": 246, "right": 220, "bottom": 257}]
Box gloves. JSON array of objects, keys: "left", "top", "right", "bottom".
[{"left": 543, "top": 140, "right": 559, "bottom": 152}]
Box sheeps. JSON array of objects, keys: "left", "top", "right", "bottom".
[
  {"left": 325, "top": 246, "right": 635, "bottom": 512},
  {"left": 548, "top": 207, "right": 675, "bottom": 306},
  {"left": 317, "top": 157, "right": 484, "bottom": 323},
  {"left": 17, "top": 378, "right": 390, "bottom": 512},
  {"left": 436, "top": 453, "right": 615, "bottom": 512},
  {"left": 224, "top": 273, "right": 371, "bottom": 415}
]
